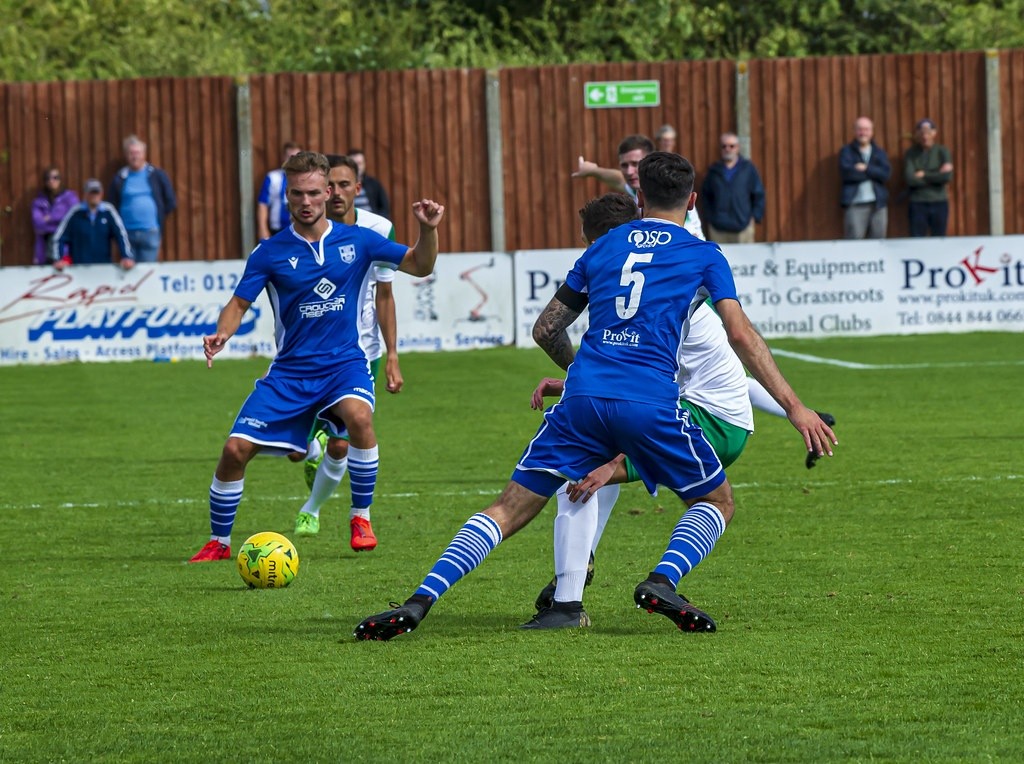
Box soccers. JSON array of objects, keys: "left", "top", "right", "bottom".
[{"left": 237, "top": 531, "right": 300, "bottom": 589}]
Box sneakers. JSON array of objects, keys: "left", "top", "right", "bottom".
[
  {"left": 805, "top": 410, "right": 835, "bottom": 469},
  {"left": 294, "top": 512, "right": 320, "bottom": 536},
  {"left": 354, "top": 601, "right": 425, "bottom": 642},
  {"left": 535, "top": 551, "right": 594, "bottom": 610},
  {"left": 634, "top": 571, "right": 717, "bottom": 633},
  {"left": 190, "top": 540, "right": 231, "bottom": 562},
  {"left": 304, "top": 430, "right": 327, "bottom": 492},
  {"left": 350, "top": 515, "right": 377, "bottom": 551},
  {"left": 522, "top": 609, "right": 587, "bottom": 629}
]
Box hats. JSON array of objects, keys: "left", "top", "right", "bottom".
[{"left": 85, "top": 180, "right": 102, "bottom": 192}]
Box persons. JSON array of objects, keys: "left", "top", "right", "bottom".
[
  {"left": 699, "top": 133, "right": 765, "bottom": 243},
  {"left": 344, "top": 149, "right": 388, "bottom": 218},
  {"left": 902, "top": 120, "right": 953, "bottom": 237},
  {"left": 521, "top": 195, "right": 755, "bottom": 630},
  {"left": 187, "top": 152, "right": 444, "bottom": 562},
  {"left": 834, "top": 117, "right": 892, "bottom": 240},
  {"left": 30, "top": 166, "right": 81, "bottom": 265},
  {"left": 353, "top": 152, "right": 839, "bottom": 641},
  {"left": 109, "top": 135, "right": 178, "bottom": 262},
  {"left": 51, "top": 180, "right": 136, "bottom": 272},
  {"left": 295, "top": 155, "right": 397, "bottom": 535},
  {"left": 569, "top": 136, "right": 705, "bottom": 239},
  {"left": 655, "top": 125, "right": 676, "bottom": 154},
  {"left": 257, "top": 142, "right": 305, "bottom": 240}
]
